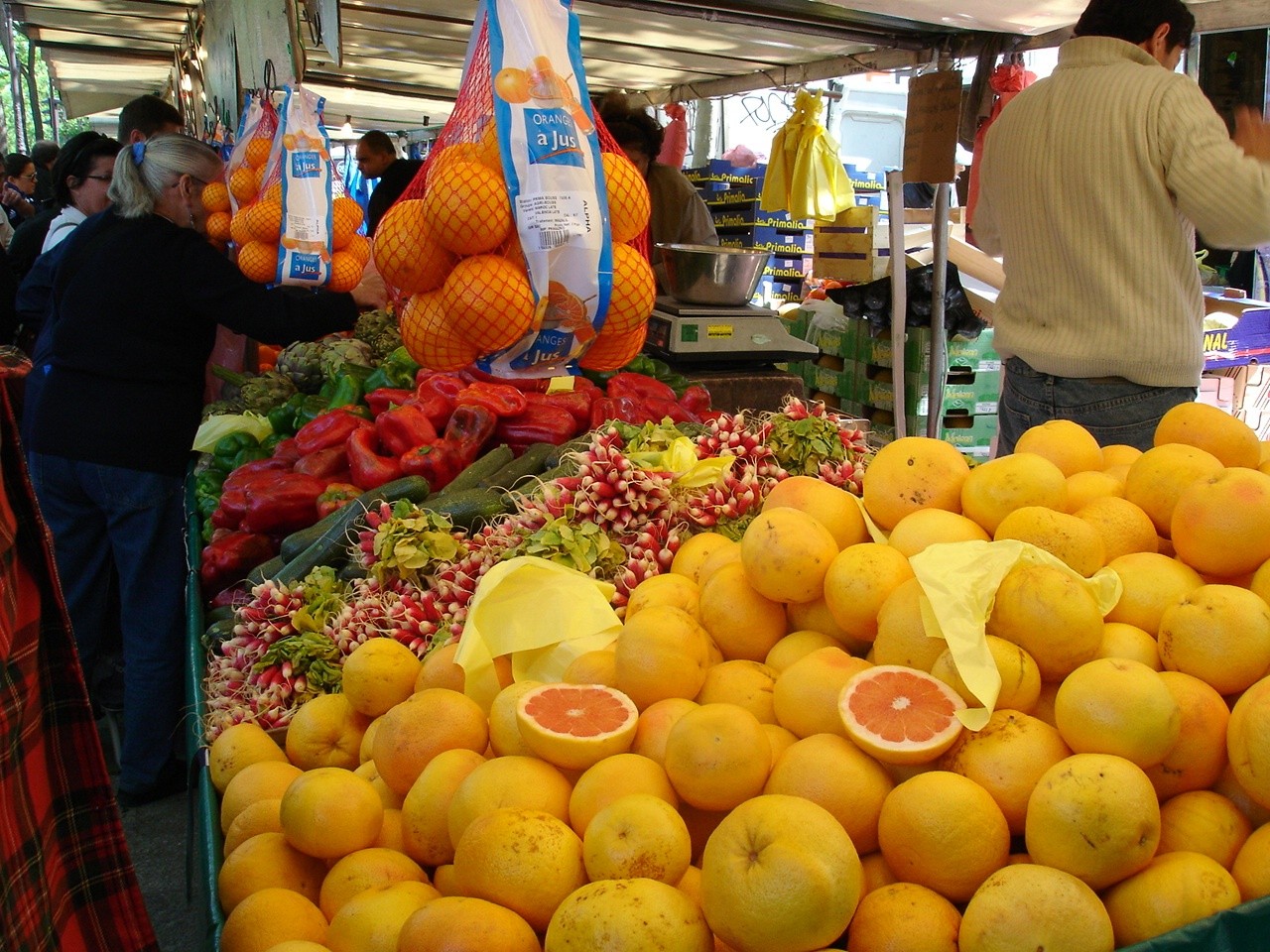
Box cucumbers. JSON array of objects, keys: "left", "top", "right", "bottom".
[{"left": 201, "top": 435, "right": 590, "bottom": 656}]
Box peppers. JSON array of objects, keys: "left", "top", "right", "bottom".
[{"left": 197, "top": 347, "right": 736, "bottom": 604}]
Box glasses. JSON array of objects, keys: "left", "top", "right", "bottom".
[
  {"left": 17, "top": 172, "right": 37, "bottom": 181},
  {"left": 75, "top": 171, "right": 113, "bottom": 183}
]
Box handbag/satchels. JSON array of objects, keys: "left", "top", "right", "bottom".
[
  {"left": 1195, "top": 249, "right": 1221, "bottom": 286},
  {"left": 0, "top": 321, "right": 32, "bottom": 405}
]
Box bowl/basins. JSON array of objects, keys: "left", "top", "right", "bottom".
[{"left": 653, "top": 242, "right": 775, "bottom": 307}]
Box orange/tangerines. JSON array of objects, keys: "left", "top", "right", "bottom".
[
  {"left": 204, "top": 134, "right": 372, "bottom": 293},
  {"left": 494, "top": 69, "right": 535, "bottom": 103},
  {"left": 208, "top": 639, "right": 578, "bottom": 951},
  {"left": 371, "top": 122, "right": 655, "bottom": 372},
  {"left": 516, "top": 400, "right": 1270, "bottom": 951},
  {"left": 808, "top": 280, "right": 842, "bottom": 301}
]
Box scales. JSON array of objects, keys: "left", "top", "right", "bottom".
[{"left": 642, "top": 241, "right": 819, "bottom": 373}]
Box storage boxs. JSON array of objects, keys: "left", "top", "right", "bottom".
[
  {"left": 1194, "top": 285, "right": 1270, "bottom": 442},
  {"left": 680, "top": 158, "right": 1015, "bottom": 463}
]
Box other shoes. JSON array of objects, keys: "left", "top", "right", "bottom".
[
  {"left": 115, "top": 749, "right": 199, "bottom": 806},
  {"left": 92, "top": 704, "right": 106, "bottom": 719}
]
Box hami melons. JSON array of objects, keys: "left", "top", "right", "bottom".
[{"left": 776, "top": 301, "right": 971, "bottom": 429}]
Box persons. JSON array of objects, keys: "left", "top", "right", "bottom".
[
  {"left": 969, "top": 0, "right": 1270, "bottom": 463},
  {"left": 0, "top": 97, "right": 391, "bottom": 808},
  {"left": 355, "top": 130, "right": 428, "bottom": 239},
  {"left": 590, "top": 93, "right": 718, "bottom": 300}
]
